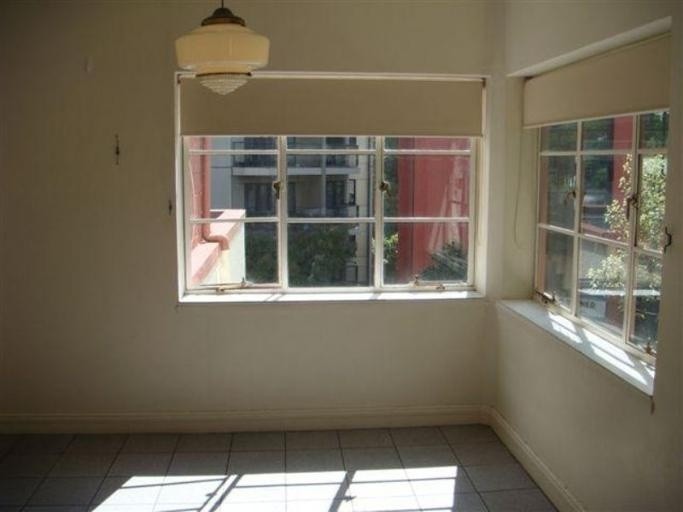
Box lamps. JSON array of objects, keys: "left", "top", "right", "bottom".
[{"left": 175, "top": 1, "right": 270, "bottom": 96}]
